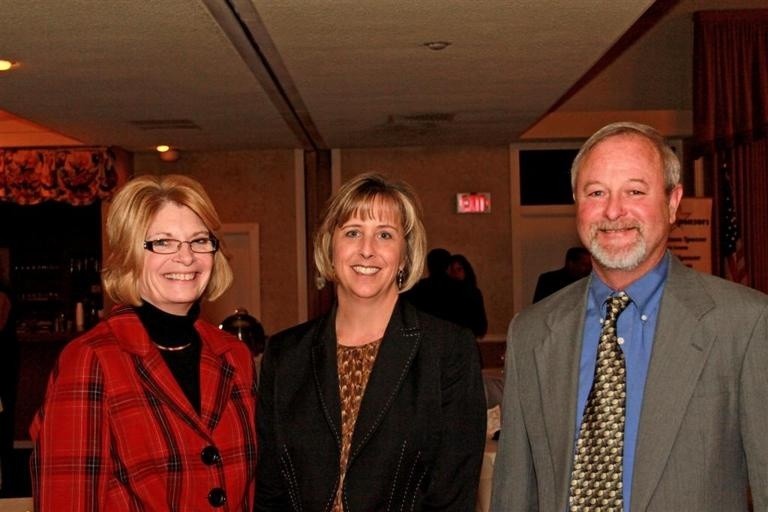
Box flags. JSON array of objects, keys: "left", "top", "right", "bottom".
[{"left": 718, "top": 154, "right": 748, "bottom": 286}]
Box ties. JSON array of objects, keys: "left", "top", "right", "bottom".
[{"left": 568, "top": 291, "right": 632, "bottom": 512}]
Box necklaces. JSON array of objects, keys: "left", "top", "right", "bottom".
[{"left": 150, "top": 340, "right": 190, "bottom": 353}]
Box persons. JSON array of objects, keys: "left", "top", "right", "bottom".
[
  {"left": 489, "top": 121, "right": 767, "bottom": 511},
  {"left": 27, "top": 171, "right": 257, "bottom": 512},
  {"left": 256, "top": 172, "right": 487, "bottom": 511},
  {"left": 414, "top": 248, "right": 595, "bottom": 336}
]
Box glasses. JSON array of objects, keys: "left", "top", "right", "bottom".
[{"left": 144, "top": 232, "right": 217, "bottom": 254}]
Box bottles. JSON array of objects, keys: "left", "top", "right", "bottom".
[
  {"left": 75, "top": 301, "right": 85, "bottom": 332},
  {"left": 67, "top": 249, "right": 101, "bottom": 292}
]
